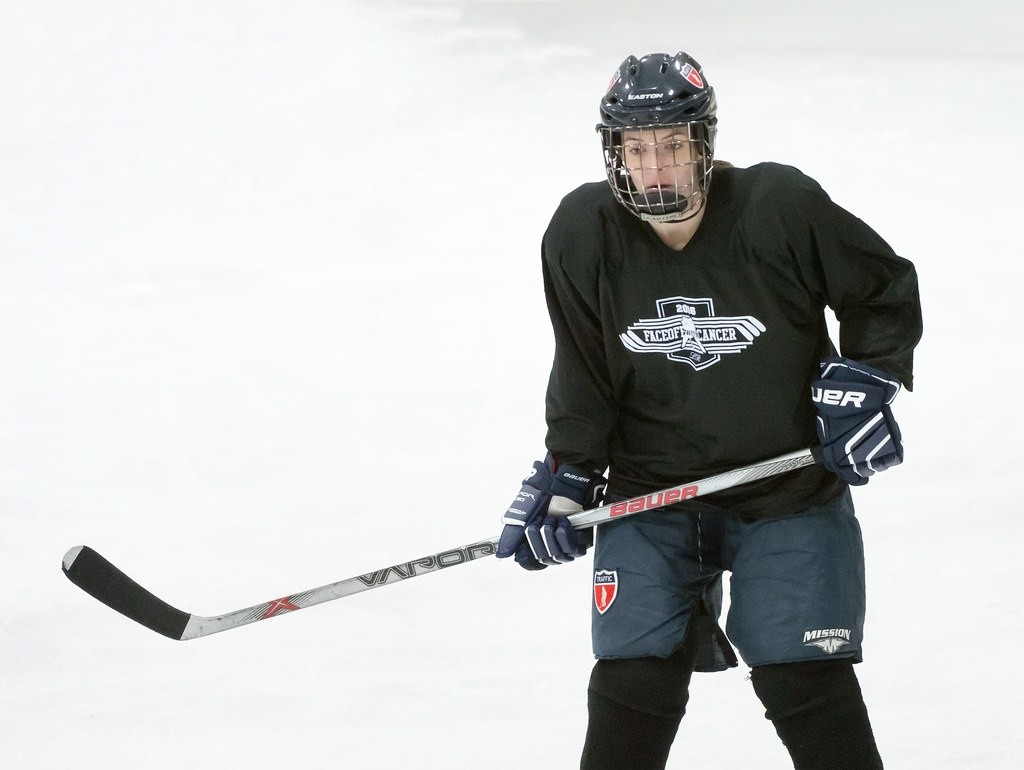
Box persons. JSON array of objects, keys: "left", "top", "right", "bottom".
[{"left": 494, "top": 52, "right": 922, "bottom": 770}]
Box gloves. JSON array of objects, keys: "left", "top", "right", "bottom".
[
  {"left": 810, "top": 357, "right": 906, "bottom": 487},
  {"left": 495, "top": 452, "right": 606, "bottom": 571}
]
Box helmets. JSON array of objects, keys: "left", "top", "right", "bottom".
[{"left": 595, "top": 54, "right": 716, "bottom": 226}]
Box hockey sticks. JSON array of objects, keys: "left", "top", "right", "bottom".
[{"left": 60, "top": 435, "right": 817, "bottom": 643}]
